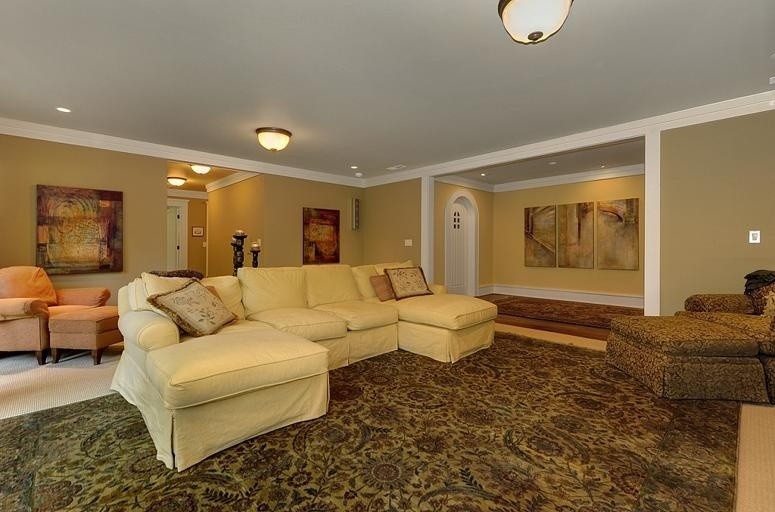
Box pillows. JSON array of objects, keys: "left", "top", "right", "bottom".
[
  {"left": 146, "top": 275, "right": 240, "bottom": 336},
  {"left": 352, "top": 260, "right": 406, "bottom": 300},
  {"left": 128, "top": 275, "right": 246, "bottom": 325},
  {"left": 374, "top": 260, "right": 414, "bottom": 275},
  {"left": 383, "top": 265, "right": 433, "bottom": 301},
  {"left": 369, "top": 275, "right": 397, "bottom": 302},
  {"left": 139, "top": 271, "right": 202, "bottom": 322},
  {"left": 236, "top": 265, "right": 309, "bottom": 320},
  {"left": 0, "top": 265, "right": 57, "bottom": 305},
  {"left": 300, "top": 261, "right": 365, "bottom": 308}
]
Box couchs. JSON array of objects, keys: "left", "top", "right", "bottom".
[
  {"left": 349, "top": 260, "right": 498, "bottom": 363},
  {"left": 110, "top": 272, "right": 332, "bottom": 471}
]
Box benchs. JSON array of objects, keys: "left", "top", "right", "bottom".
[
  {"left": 49, "top": 304, "right": 121, "bottom": 365},
  {"left": 604, "top": 315, "right": 771, "bottom": 403}
]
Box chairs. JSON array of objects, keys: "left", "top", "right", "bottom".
[
  {"left": 236, "top": 259, "right": 348, "bottom": 375},
  {"left": 301, "top": 265, "right": 398, "bottom": 363},
  {"left": 673, "top": 271, "right": 775, "bottom": 405},
  {"left": 0, "top": 333, "right": 743, "bottom": 512},
  {"left": 0, "top": 266, "right": 111, "bottom": 365}
]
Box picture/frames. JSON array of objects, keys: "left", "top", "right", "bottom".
[{"left": 192, "top": 227, "right": 204, "bottom": 236}]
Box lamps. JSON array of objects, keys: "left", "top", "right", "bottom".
[
  {"left": 167, "top": 176, "right": 187, "bottom": 187},
  {"left": 190, "top": 164, "right": 211, "bottom": 174},
  {"left": 497, "top": 0, "right": 575, "bottom": 44},
  {"left": 255, "top": 127, "right": 293, "bottom": 153}
]
